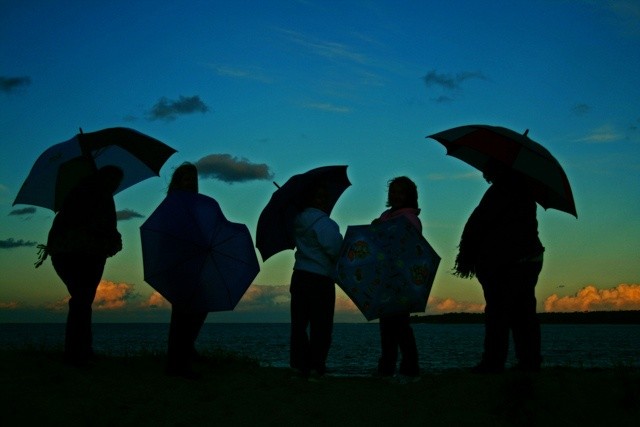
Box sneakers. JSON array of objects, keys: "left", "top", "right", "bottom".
[
  {"left": 307, "top": 371, "right": 329, "bottom": 383},
  {"left": 395, "top": 374, "right": 420, "bottom": 385},
  {"left": 291, "top": 368, "right": 306, "bottom": 381}
]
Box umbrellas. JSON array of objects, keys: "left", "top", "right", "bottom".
[
  {"left": 255, "top": 164, "right": 355, "bottom": 263},
  {"left": 426, "top": 123, "right": 578, "bottom": 220},
  {"left": 335, "top": 217, "right": 440, "bottom": 321},
  {"left": 13, "top": 127, "right": 179, "bottom": 214},
  {"left": 140, "top": 190, "right": 260, "bottom": 313}
]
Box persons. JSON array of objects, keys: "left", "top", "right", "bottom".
[
  {"left": 34, "top": 195, "right": 123, "bottom": 368},
  {"left": 166, "top": 162, "right": 210, "bottom": 379},
  {"left": 367, "top": 176, "right": 422, "bottom": 376},
  {"left": 451, "top": 151, "right": 546, "bottom": 375},
  {"left": 286, "top": 180, "right": 346, "bottom": 384}
]
